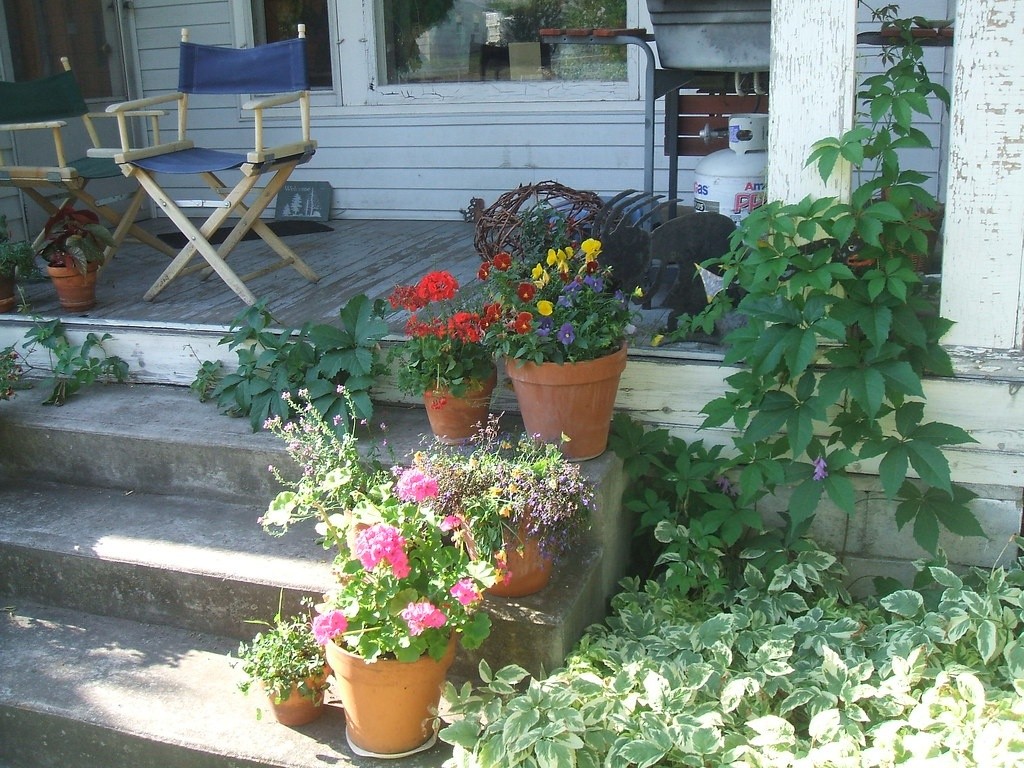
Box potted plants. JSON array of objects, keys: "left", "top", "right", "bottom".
[
  {"left": 43, "top": 206, "right": 118, "bottom": 312},
  {"left": 226, "top": 588, "right": 332, "bottom": 726},
  {"left": 0, "top": 214, "right": 44, "bottom": 314}
]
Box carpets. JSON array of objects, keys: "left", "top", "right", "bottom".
[{"left": 157, "top": 219, "right": 335, "bottom": 250}]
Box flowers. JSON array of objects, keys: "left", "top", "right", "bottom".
[
  {"left": 417, "top": 410, "right": 598, "bottom": 572},
  {"left": 478, "top": 237, "right": 644, "bottom": 369},
  {"left": 389, "top": 270, "right": 497, "bottom": 409},
  {"left": 312, "top": 466, "right": 498, "bottom": 666}
]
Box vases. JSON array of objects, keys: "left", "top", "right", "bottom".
[
  {"left": 504, "top": 339, "right": 628, "bottom": 464},
  {"left": 459, "top": 503, "right": 557, "bottom": 599},
  {"left": 421, "top": 360, "right": 497, "bottom": 446},
  {"left": 326, "top": 627, "right": 457, "bottom": 755}
]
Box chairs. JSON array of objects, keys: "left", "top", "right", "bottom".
[
  {"left": 0, "top": 55, "right": 180, "bottom": 273},
  {"left": 468, "top": 32, "right": 551, "bottom": 82},
  {"left": 105, "top": 24, "right": 317, "bottom": 313}
]
